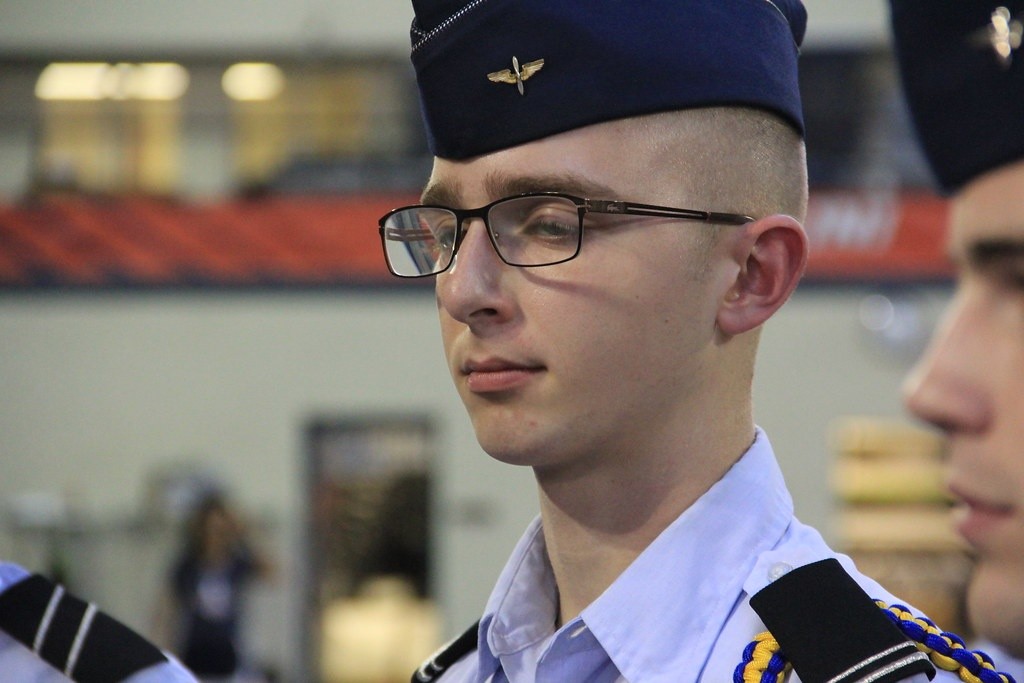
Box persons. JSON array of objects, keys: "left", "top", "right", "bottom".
[
  {"left": 0, "top": 559, "right": 200, "bottom": 683},
  {"left": 377, "top": 0, "right": 1020, "bottom": 683},
  {"left": 889, "top": 1, "right": 1024, "bottom": 658},
  {"left": 139, "top": 489, "right": 281, "bottom": 683}
]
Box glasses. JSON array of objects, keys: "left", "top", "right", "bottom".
[{"left": 378, "top": 192, "right": 758, "bottom": 280}]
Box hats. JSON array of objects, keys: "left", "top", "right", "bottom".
[
  {"left": 410, "top": 0, "right": 807, "bottom": 163},
  {"left": 888, "top": 0, "right": 1024, "bottom": 199}
]
